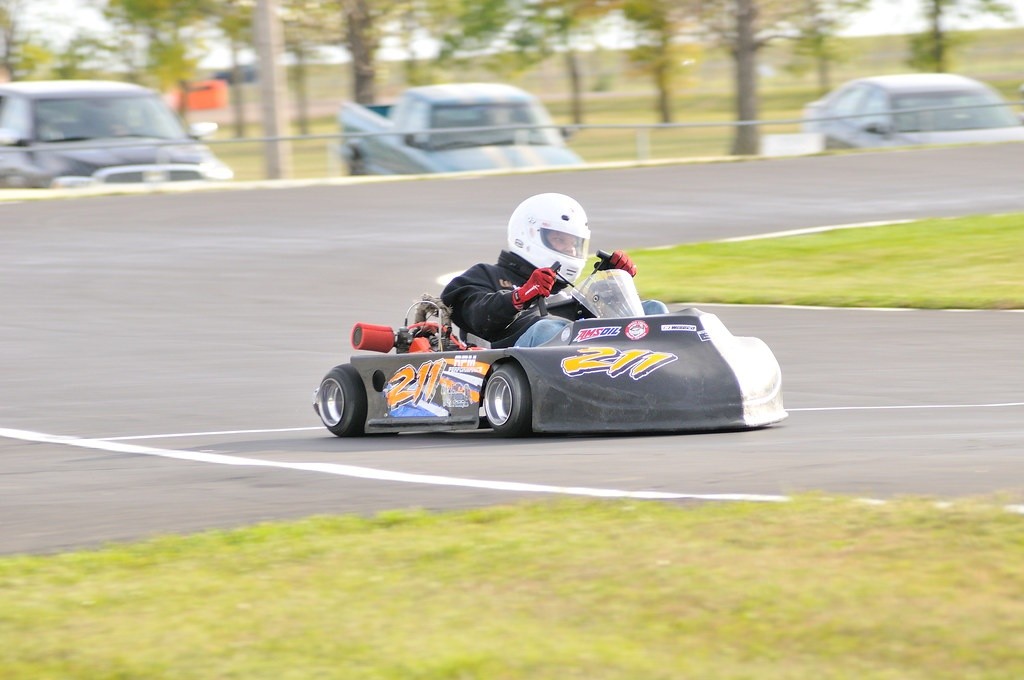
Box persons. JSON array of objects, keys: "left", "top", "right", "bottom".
[{"left": 440, "top": 194, "right": 668, "bottom": 348}]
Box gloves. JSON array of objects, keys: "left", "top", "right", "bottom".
[
  {"left": 594, "top": 249, "right": 637, "bottom": 278},
  {"left": 512, "top": 267, "right": 557, "bottom": 312}
]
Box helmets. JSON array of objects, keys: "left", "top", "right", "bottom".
[{"left": 507, "top": 192, "right": 592, "bottom": 283}]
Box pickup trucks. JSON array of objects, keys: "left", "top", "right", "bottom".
[{"left": 333, "top": 82, "right": 582, "bottom": 174}]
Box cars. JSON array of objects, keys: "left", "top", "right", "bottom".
[
  {"left": 0, "top": 77, "right": 234, "bottom": 190},
  {"left": 806, "top": 72, "right": 1023, "bottom": 146}
]
